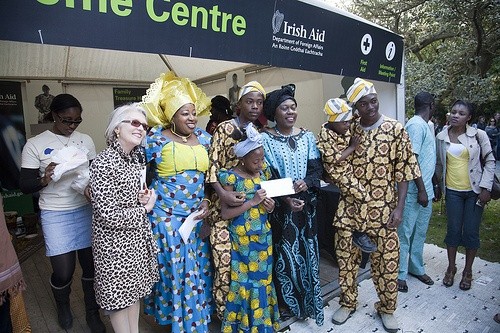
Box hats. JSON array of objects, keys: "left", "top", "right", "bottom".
[
  {"left": 210, "top": 95, "right": 233, "bottom": 116},
  {"left": 346, "top": 76, "right": 377, "bottom": 106},
  {"left": 141, "top": 70, "right": 212, "bottom": 127},
  {"left": 238, "top": 81, "right": 266, "bottom": 103},
  {"left": 263, "top": 86, "right": 297, "bottom": 123},
  {"left": 323, "top": 98, "right": 353, "bottom": 123}
]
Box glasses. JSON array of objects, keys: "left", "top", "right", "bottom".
[
  {"left": 490, "top": 121, "right": 495, "bottom": 122},
  {"left": 121, "top": 119, "right": 149, "bottom": 131},
  {"left": 431, "top": 93, "right": 436, "bottom": 103},
  {"left": 55, "top": 111, "right": 83, "bottom": 126}
]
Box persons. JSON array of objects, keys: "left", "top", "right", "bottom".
[
  {"left": 467, "top": 112, "right": 500, "bottom": 161},
  {"left": 316, "top": 76, "right": 422, "bottom": 333},
  {"left": 141, "top": 73, "right": 217, "bottom": 333},
  {"left": 435, "top": 100, "right": 496, "bottom": 289},
  {"left": 427, "top": 112, "right": 451, "bottom": 136},
  {"left": 397, "top": 91, "right": 437, "bottom": 293},
  {"left": 17, "top": 93, "right": 106, "bottom": 333},
  {"left": 255, "top": 82, "right": 324, "bottom": 328},
  {"left": 90, "top": 106, "right": 161, "bottom": 333},
  {"left": 205, "top": 81, "right": 281, "bottom": 333}
]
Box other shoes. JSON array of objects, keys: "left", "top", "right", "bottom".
[
  {"left": 377, "top": 308, "right": 398, "bottom": 333},
  {"left": 408, "top": 270, "right": 434, "bottom": 285},
  {"left": 332, "top": 305, "right": 356, "bottom": 324},
  {"left": 397, "top": 279, "right": 408, "bottom": 293}
]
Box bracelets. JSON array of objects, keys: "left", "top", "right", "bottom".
[
  {"left": 203, "top": 198, "right": 212, "bottom": 206},
  {"left": 142, "top": 206, "right": 147, "bottom": 214},
  {"left": 434, "top": 185, "right": 439, "bottom": 188},
  {"left": 267, "top": 209, "right": 274, "bottom": 213}
]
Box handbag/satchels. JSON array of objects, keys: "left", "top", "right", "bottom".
[
  {"left": 474, "top": 129, "right": 499, "bottom": 200},
  {"left": 492, "top": 135, "right": 498, "bottom": 146}
]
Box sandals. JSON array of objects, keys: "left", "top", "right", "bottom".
[
  {"left": 442, "top": 266, "right": 457, "bottom": 287},
  {"left": 459, "top": 270, "right": 472, "bottom": 290}
]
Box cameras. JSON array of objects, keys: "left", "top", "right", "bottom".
[{"left": 476, "top": 199, "right": 483, "bottom": 207}]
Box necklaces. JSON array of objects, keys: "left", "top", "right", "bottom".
[
  {"left": 56, "top": 134, "right": 72, "bottom": 147},
  {"left": 237, "top": 166, "right": 253, "bottom": 179},
  {"left": 170, "top": 127, "right": 191, "bottom": 143},
  {"left": 273, "top": 126, "right": 294, "bottom": 137}
]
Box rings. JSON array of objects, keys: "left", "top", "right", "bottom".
[{"left": 300, "top": 185, "right": 302, "bottom": 188}]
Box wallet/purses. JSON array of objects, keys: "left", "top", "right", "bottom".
[{"left": 200, "top": 221, "right": 211, "bottom": 239}]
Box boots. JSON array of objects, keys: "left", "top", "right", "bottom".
[
  {"left": 80, "top": 276, "right": 106, "bottom": 333},
  {"left": 49, "top": 273, "right": 74, "bottom": 330}
]
